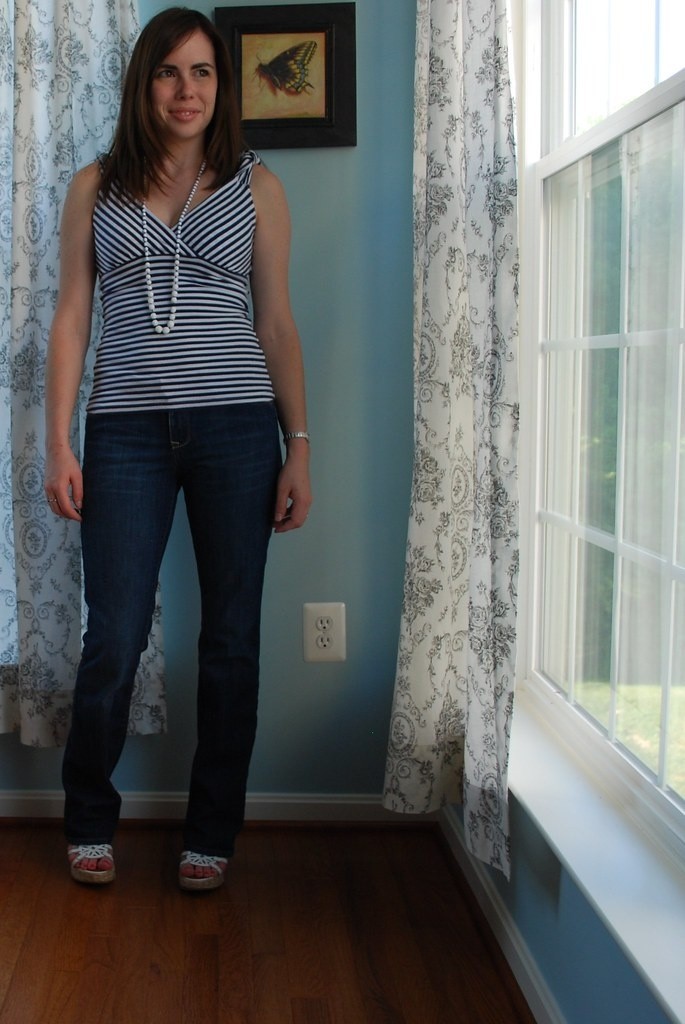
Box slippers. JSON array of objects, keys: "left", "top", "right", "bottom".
[
  {"left": 66, "top": 844, "right": 116, "bottom": 884},
  {"left": 177, "top": 850, "right": 228, "bottom": 890}
]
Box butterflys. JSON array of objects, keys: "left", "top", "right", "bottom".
[{"left": 252, "top": 40, "right": 318, "bottom": 96}]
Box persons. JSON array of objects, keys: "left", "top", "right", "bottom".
[{"left": 43, "top": 7, "right": 312, "bottom": 890}]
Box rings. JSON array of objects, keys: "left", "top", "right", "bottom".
[
  {"left": 306, "top": 514, "right": 309, "bottom": 518},
  {"left": 49, "top": 499, "right": 57, "bottom": 502}
]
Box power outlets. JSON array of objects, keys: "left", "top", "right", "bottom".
[{"left": 303, "top": 602, "right": 347, "bottom": 663}]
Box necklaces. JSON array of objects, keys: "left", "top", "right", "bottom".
[{"left": 142, "top": 155, "right": 208, "bottom": 334}]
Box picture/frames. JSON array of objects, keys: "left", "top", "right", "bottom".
[{"left": 214, "top": 1, "right": 358, "bottom": 150}]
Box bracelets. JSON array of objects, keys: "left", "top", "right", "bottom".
[{"left": 283, "top": 432, "right": 310, "bottom": 443}]
